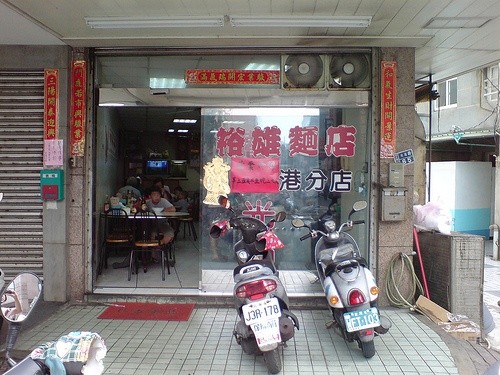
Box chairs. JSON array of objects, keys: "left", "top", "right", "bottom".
[{"left": 98, "top": 194, "right": 200, "bottom": 281}]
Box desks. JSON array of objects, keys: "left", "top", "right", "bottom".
[{"left": 101, "top": 211, "right": 190, "bottom": 264}]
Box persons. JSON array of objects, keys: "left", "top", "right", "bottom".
[
  {"left": 119, "top": 177, "right": 141, "bottom": 197},
  {"left": 154, "top": 177, "right": 171, "bottom": 199},
  {"left": 172, "top": 190, "right": 190, "bottom": 213},
  {"left": 1, "top": 291, "right": 22, "bottom": 317},
  {"left": 146, "top": 186, "right": 176, "bottom": 263}
]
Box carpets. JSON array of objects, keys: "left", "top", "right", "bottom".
[{"left": 97, "top": 302, "right": 195, "bottom": 321}]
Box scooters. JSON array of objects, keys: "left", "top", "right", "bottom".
[
  {"left": 291, "top": 200, "right": 391, "bottom": 357},
  {"left": 210, "top": 194, "right": 301, "bottom": 373},
  {"left": 0, "top": 269, "right": 107, "bottom": 374}
]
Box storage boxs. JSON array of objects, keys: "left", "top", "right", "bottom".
[
  {"left": 171, "top": 160, "right": 187, "bottom": 178},
  {"left": 146, "top": 159, "right": 169, "bottom": 176}
]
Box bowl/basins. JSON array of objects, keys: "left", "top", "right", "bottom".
[{"left": 152, "top": 208, "right": 164, "bottom": 214}]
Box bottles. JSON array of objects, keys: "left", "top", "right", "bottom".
[
  {"left": 141, "top": 192, "right": 147, "bottom": 213},
  {"left": 104, "top": 195, "right": 110, "bottom": 213},
  {"left": 127, "top": 192, "right": 131, "bottom": 205}
]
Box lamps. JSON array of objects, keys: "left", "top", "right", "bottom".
[
  {"left": 84, "top": 16, "right": 224, "bottom": 29},
  {"left": 230, "top": 16, "right": 372, "bottom": 28}
]
